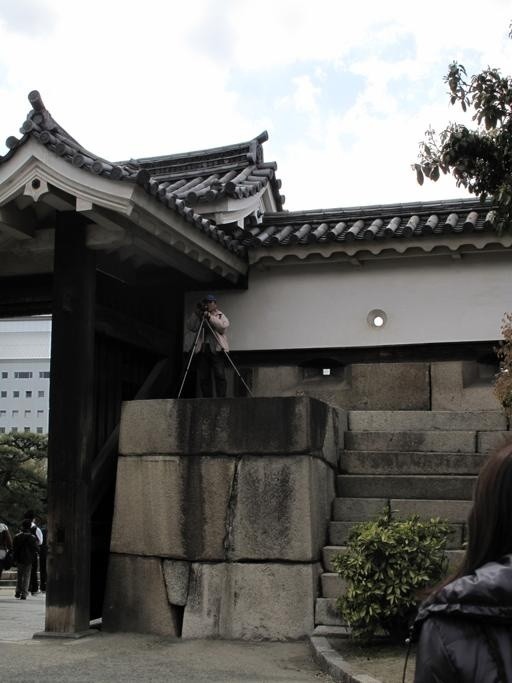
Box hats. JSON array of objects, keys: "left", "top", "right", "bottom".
[{"left": 207, "top": 294, "right": 216, "bottom": 301}]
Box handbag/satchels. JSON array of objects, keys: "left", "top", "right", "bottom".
[{"left": 3, "top": 550, "right": 14, "bottom": 569}]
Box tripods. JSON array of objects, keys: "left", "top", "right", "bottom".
[{"left": 177, "top": 317, "right": 257, "bottom": 399}]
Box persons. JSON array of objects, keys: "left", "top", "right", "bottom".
[
  {"left": 38, "top": 523, "right": 48, "bottom": 590},
  {"left": 10, "top": 519, "right": 42, "bottom": 599},
  {"left": 19, "top": 510, "right": 44, "bottom": 597},
  {"left": 0, "top": 519, "right": 12, "bottom": 581},
  {"left": 187, "top": 294, "right": 231, "bottom": 397},
  {"left": 408, "top": 442, "right": 512, "bottom": 683}
]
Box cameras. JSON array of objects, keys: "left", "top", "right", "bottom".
[{"left": 195, "top": 300, "right": 209, "bottom": 317}]
